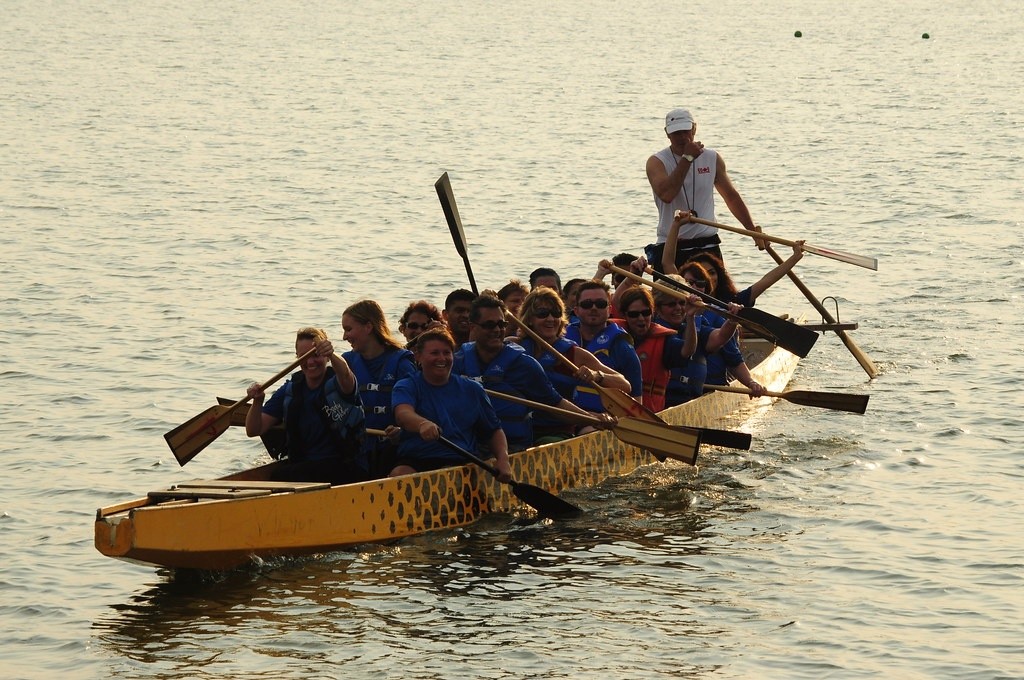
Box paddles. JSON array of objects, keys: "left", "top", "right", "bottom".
[
  {"left": 482, "top": 388, "right": 702, "bottom": 467},
  {"left": 672, "top": 208, "right": 880, "bottom": 272},
  {"left": 641, "top": 263, "right": 821, "bottom": 361},
  {"left": 606, "top": 264, "right": 783, "bottom": 348},
  {"left": 437, "top": 433, "right": 588, "bottom": 522},
  {"left": 701, "top": 381, "right": 871, "bottom": 414},
  {"left": 162, "top": 334, "right": 329, "bottom": 468},
  {"left": 678, "top": 424, "right": 753, "bottom": 452},
  {"left": 215, "top": 395, "right": 401, "bottom": 440},
  {"left": 503, "top": 305, "right": 669, "bottom": 464},
  {"left": 434, "top": 169, "right": 483, "bottom": 298},
  {"left": 760, "top": 239, "right": 883, "bottom": 381}
]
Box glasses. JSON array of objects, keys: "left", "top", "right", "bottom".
[
  {"left": 686, "top": 279, "right": 708, "bottom": 289},
  {"left": 623, "top": 309, "right": 652, "bottom": 318},
  {"left": 405, "top": 321, "right": 429, "bottom": 331},
  {"left": 577, "top": 300, "right": 610, "bottom": 310},
  {"left": 614, "top": 274, "right": 626, "bottom": 284},
  {"left": 531, "top": 307, "right": 563, "bottom": 319},
  {"left": 471, "top": 320, "right": 509, "bottom": 331},
  {"left": 659, "top": 300, "right": 686, "bottom": 308}
]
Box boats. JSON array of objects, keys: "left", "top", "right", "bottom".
[{"left": 92, "top": 316, "right": 802, "bottom": 573}]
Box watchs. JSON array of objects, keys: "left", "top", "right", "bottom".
[
  {"left": 682, "top": 154, "right": 694, "bottom": 163},
  {"left": 595, "top": 370, "right": 604, "bottom": 385}
]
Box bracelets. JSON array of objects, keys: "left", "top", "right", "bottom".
[{"left": 418, "top": 420, "right": 430, "bottom": 427}]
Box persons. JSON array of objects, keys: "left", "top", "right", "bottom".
[
  {"left": 244, "top": 327, "right": 367, "bottom": 484},
  {"left": 386, "top": 327, "right": 513, "bottom": 481},
  {"left": 646, "top": 108, "right": 771, "bottom": 283},
  {"left": 336, "top": 209, "right": 806, "bottom": 453}
]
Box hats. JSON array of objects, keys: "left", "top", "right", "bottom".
[{"left": 665, "top": 109, "right": 695, "bottom": 135}]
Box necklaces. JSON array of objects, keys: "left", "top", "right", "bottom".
[{"left": 669, "top": 144, "right": 697, "bottom": 224}]
